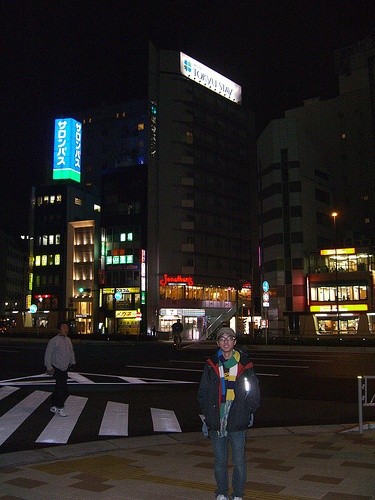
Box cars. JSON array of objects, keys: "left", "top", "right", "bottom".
[{"left": 0, "top": 319, "right": 17, "bottom": 335}]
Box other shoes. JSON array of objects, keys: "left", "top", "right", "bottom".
[
  {"left": 50, "top": 406, "right": 59, "bottom": 415},
  {"left": 216, "top": 494, "right": 242, "bottom": 500},
  {"left": 57, "top": 408, "right": 67, "bottom": 416}
]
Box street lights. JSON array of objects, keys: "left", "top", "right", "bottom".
[{"left": 331, "top": 212, "right": 340, "bottom": 339}]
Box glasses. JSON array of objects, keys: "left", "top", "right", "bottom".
[{"left": 218, "top": 336, "right": 234, "bottom": 341}]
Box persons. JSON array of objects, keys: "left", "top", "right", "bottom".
[
  {"left": 171, "top": 319, "right": 183, "bottom": 344},
  {"left": 198, "top": 327, "right": 260, "bottom": 500},
  {"left": 44, "top": 321, "right": 75, "bottom": 417}
]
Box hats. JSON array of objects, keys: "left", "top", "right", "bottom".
[{"left": 217, "top": 327, "right": 235, "bottom": 337}]
